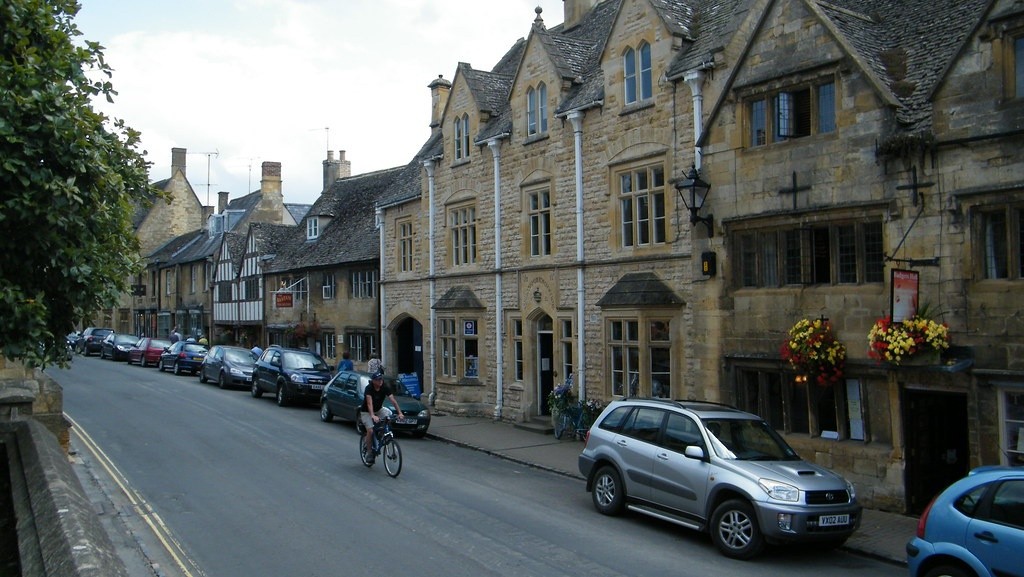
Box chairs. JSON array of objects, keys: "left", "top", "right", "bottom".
[{"left": 708, "top": 422, "right": 721, "bottom": 439}]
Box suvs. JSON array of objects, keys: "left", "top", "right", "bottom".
[
  {"left": 578, "top": 396, "right": 864, "bottom": 561},
  {"left": 251, "top": 345, "right": 335, "bottom": 407}
]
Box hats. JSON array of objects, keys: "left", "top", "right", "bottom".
[
  {"left": 371, "top": 372, "right": 383, "bottom": 380},
  {"left": 200, "top": 335, "right": 206, "bottom": 338},
  {"left": 343, "top": 351, "right": 350, "bottom": 358}
]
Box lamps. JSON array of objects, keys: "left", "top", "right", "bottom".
[{"left": 675, "top": 162, "right": 713, "bottom": 238}]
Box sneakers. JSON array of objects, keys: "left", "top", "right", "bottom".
[{"left": 366, "top": 454, "right": 375, "bottom": 464}]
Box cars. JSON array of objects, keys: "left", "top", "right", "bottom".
[
  {"left": 127, "top": 337, "right": 172, "bottom": 367},
  {"left": 75, "top": 327, "right": 116, "bottom": 357},
  {"left": 905, "top": 466, "right": 1024, "bottom": 577},
  {"left": 320, "top": 370, "right": 431, "bottom": 439},
  {"left": 100, "top": 334, "right": 139, "bottom": 362},
  {"left": 199, "top": 345, "right": 258, "bottom": 390},
  {"left": 158, "top": 341, "right": 211, "bottom": 375},
  {"left": 65, "top": 335, "right": 79, "bottom": 351}
]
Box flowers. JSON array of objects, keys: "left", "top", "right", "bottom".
[
  {"left": 781, "top": 319, "right": 846, "bottom": 386},
  {"left": 868, "top": 299, "right": 951, "bottom": 366},
  {"left": 548, "top": 373, "right": 608, "bottom": 426},
  {"left": 285, "top": 320, "right": 321, "bottom": 341}
]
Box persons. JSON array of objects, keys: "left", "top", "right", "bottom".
[
  {"left": 250, "top": 343, "right": 263, "bottom": 358},
  {"left": 337, "top": 351, "right": 354, "bottom": 374},
  {"left": 199, "top": 335, "right": 208, "bottom": 346},
  {"left": 360, "top": 372, "right": 404, "bottom": 463},
  {"left": 186, "top": 335, "right": 196, "bottom": 342},
  {"left": 367, "top": 353, "right": 383, "bottom": 373},
  {"left": 169, "top": 330, "right": 183, "bottom": 344}
]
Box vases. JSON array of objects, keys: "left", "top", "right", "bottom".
[{"left": 551, "top": 406, "right": 559, "bottom": 425}]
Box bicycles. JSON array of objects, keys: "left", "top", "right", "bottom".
[{"left": 358, "top": 413, "right": 403, "bottom": 478}]
